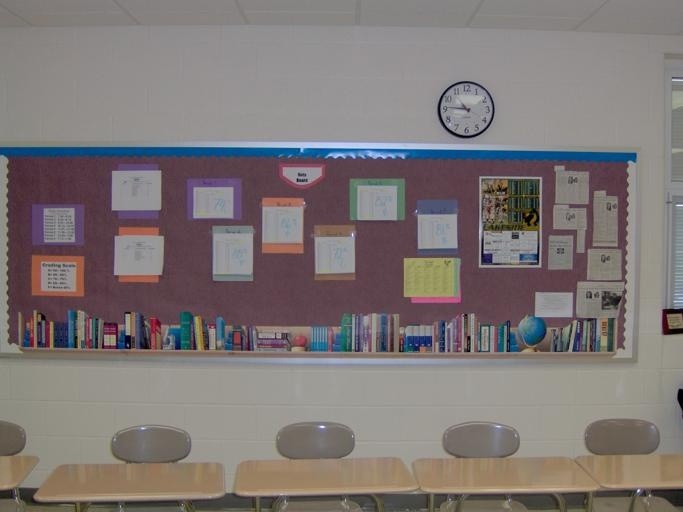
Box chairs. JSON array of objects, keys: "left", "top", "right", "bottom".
[
  {"left": 439, "top": 421, "right": 528, "bottom": 512},
  {"left": 272, "top": 422, "right": 362, "bottom": 512},
  {"left": 583, "top": 419, "right": 680, "bottom": 512},
  {"left": 111, "top": 424, "right": 191, "bottom": 512},
  {"left": 0, "top": 419, "right": 26, "bottom": 512}
]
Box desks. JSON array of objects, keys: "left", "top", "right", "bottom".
[
  {"left": 413, "top": 457, "right": 600, "bottom": 512},
  {"left": 34, "top": 463, "right": 226, "bottom": 512},
  {"left": 0, "top": 455, "right": 40, "bottom": 491},
  {"left": 234, "top": 458, "right": 419, "bottom": 512},
  {"left": 575, "top": 454, "right": 683, "bottom": 512}
]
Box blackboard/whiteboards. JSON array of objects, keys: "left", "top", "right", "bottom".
[{"left": 0, "top": 140, "right": 641, "bottom": 365}]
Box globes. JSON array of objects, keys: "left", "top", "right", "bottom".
[{"left": 517, "top": 314, "right": 547, "bottom": 352}]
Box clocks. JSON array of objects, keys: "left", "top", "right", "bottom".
[{"left": 438, "top": 80, "right": 494, "bottom": 138}]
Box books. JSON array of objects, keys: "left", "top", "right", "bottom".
[
  {"left": 550, "top": 317, "right": 616, "bottom": 353},
  {"left": 311, "top": 311, "right": 511, "bottom": 352},
  {"left": 19, "top": 311, "right": 290, "bottom": 351}
]
